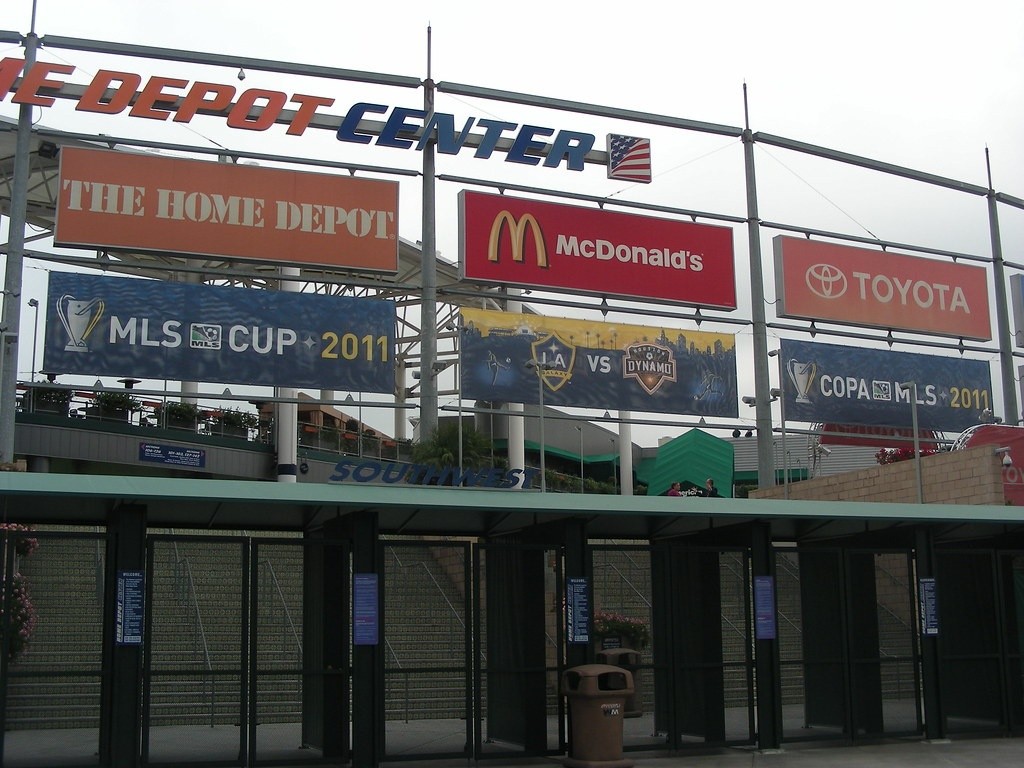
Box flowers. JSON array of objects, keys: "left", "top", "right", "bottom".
[
  {"left": 875, "top": 448, "right": 933, "bottom": 467},
  {"left": 0, "top": 523, "right": 39, "bottom": 556},
  {"left": 0, "top": 572, "right": 39, "bottom": 662},
  {"left": 593, "top": 609, "right": 648, "bottom": 645}
]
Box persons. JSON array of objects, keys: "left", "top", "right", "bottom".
[
  {"left": 667, "top": 481, "right": 683, "bottom": 497},
  {"left": 692, "top": 478, "right": 718, "bottom": 497}
]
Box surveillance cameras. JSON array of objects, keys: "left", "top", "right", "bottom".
[{"left": 1003, "top": 455, "right": 1013, "bottom": 467}]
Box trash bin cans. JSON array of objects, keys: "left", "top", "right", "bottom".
[
  {"left": 561, "top": 664, "right": 634, "bottom": 768},
  {"left": 596, "top": 648, "right": 643, "bottom": 716}
]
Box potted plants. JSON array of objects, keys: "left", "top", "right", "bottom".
[
  {"left": 153, "top": 401, "right": 202, "bottom": 433},
  {"left": 211, "top": 410, "right": 257, "bottom": 439},
  {"left": 21, "top": 388, "right": 72, "bottom": 419},
  {"left": 86, "top": 392, "right": 139, "bottom": 424},
  {"left": 312, "top": 431, "right": 336, "bottom": 452},
  {"left": 362, "top": 440, "right": 378, "bottom": 457},
  {"left": 396, "top": 447, "right": 413, "bottom": 463}
]
